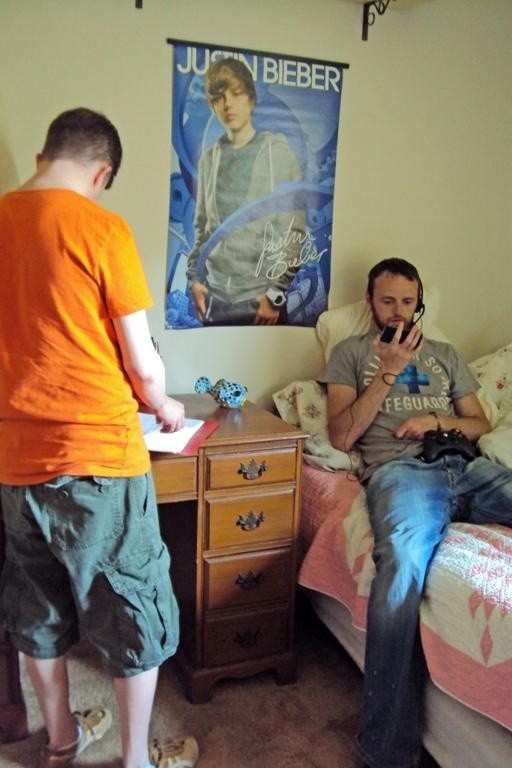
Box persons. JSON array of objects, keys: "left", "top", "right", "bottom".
[
  {"left": 185, "top": 56, "right": 309, "bottom": 326},
  {"left": 316, "top": 254, "right": 512, "bottom": 768},
  {"left": 0, "top": 105, "right": 203, "bottom": 768}
]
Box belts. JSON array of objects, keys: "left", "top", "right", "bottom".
[{"left": 208, "top": 297, "right": 255, "bottom": 318}]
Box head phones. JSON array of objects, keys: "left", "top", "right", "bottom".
[{"left": 415, "top": 277, "right": 423, "bottom": 313}]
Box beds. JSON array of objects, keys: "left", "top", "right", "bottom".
[{"left": 296, "top": 459, "right": 512, "bottom": 768}]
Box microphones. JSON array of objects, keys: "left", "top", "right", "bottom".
[{"left": 407, "top": 304, "right": 424, "bottom": 329}]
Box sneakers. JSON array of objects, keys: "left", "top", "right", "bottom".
[
  {"left": 147, "top": 735, "right": 200, "bottom": 768},
  {"left": 36, "top": 703, "right": 113, "bottom": 768}
]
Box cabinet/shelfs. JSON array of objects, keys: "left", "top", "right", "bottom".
[{"left": 133, "top": 389, "right": 312, "bottom": 704}]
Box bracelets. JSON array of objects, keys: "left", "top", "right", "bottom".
[{"left": 428, "top": 411, "right": 442, "bottom": 432}]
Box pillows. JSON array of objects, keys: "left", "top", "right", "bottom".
[{"left": 316, "top": 299, "right": 503, "bottom": 436}]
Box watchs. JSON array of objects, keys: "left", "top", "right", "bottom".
[{"left": 264, "top": 288, "right": 287, "bottom": 307}]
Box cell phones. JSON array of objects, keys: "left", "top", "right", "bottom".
[{"left": 380, "top": 326, "right": 423, "bottom": 350}]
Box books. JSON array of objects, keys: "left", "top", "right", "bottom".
[{"left": 136, "top": 408, "right": 221, "bottom": 458}]
